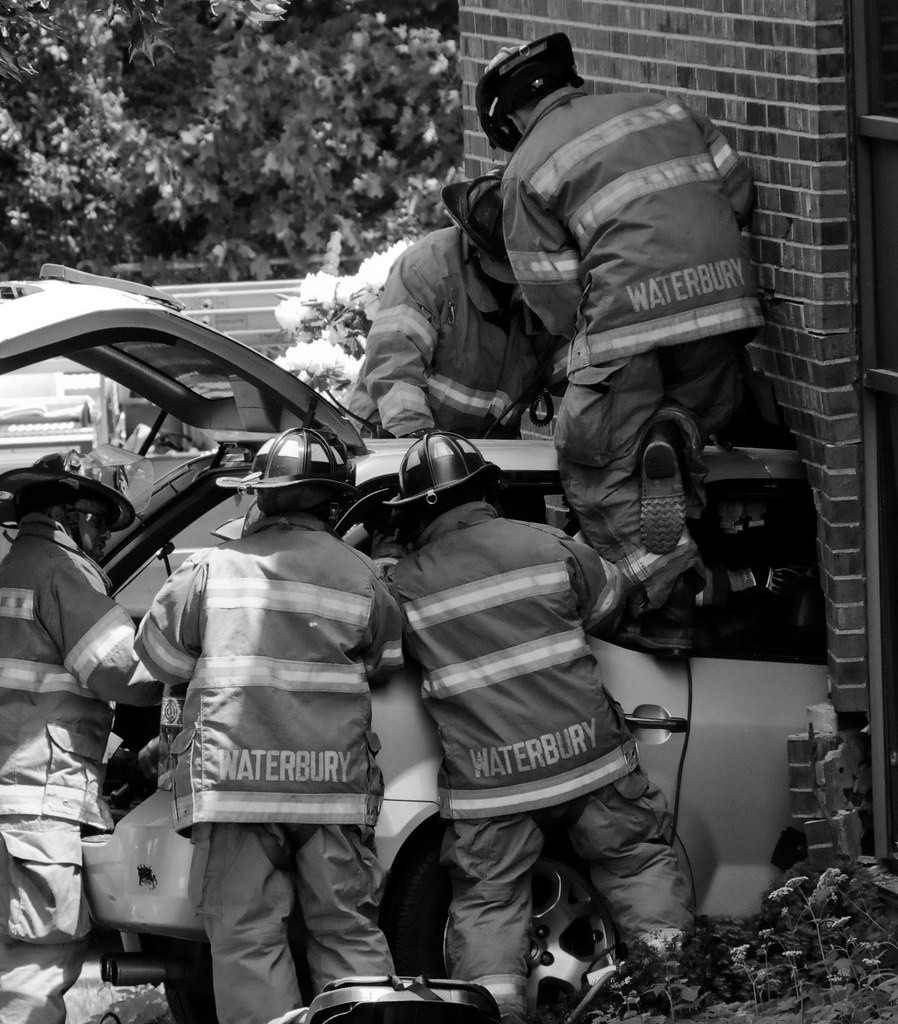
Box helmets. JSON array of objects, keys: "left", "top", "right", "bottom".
[
  {"left": 474, "top": 32, "right": 584, "bottom": 152},
  {"left": 0, "top": 453, "right": 135, "bottom": 532},
  {"left": 215, "top": 426, "right": 362, "bottom": 493},
  {"left": 382, "top": 430, "right": 501, "bottom": 505},
  {"left": 439, "top": 174, "right": 510, "bottom": 265}
]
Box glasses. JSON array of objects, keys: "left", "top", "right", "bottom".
[{"left": 62, "top": 505, "right": 112, "bottom": 525}]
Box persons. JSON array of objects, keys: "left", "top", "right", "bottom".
[
  {"left": 377, "top": 428, "right": 692, "bottom": 1023},
  {"left": 479, "top": 30, "right": 770, "bottom": 667},
  {"left": 0, "top": 466, "right": 140, "bottom": 1024},
  {"left": 138, "top": 425, "right": 405, "bottom": 1024},
  {"left": 343, "top": 170, "right": 556, "bottom": 440}
]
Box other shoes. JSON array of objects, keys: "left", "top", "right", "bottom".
[
  {"left": 639, "top": 434, "right": 687, "bottom": 556},
  {"left": 621, "top": 608, "right": 695, "bottom": 651}
]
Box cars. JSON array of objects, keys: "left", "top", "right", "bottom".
[{"left": 1, "top": 261, "right": 845, "bottom": 993}]
